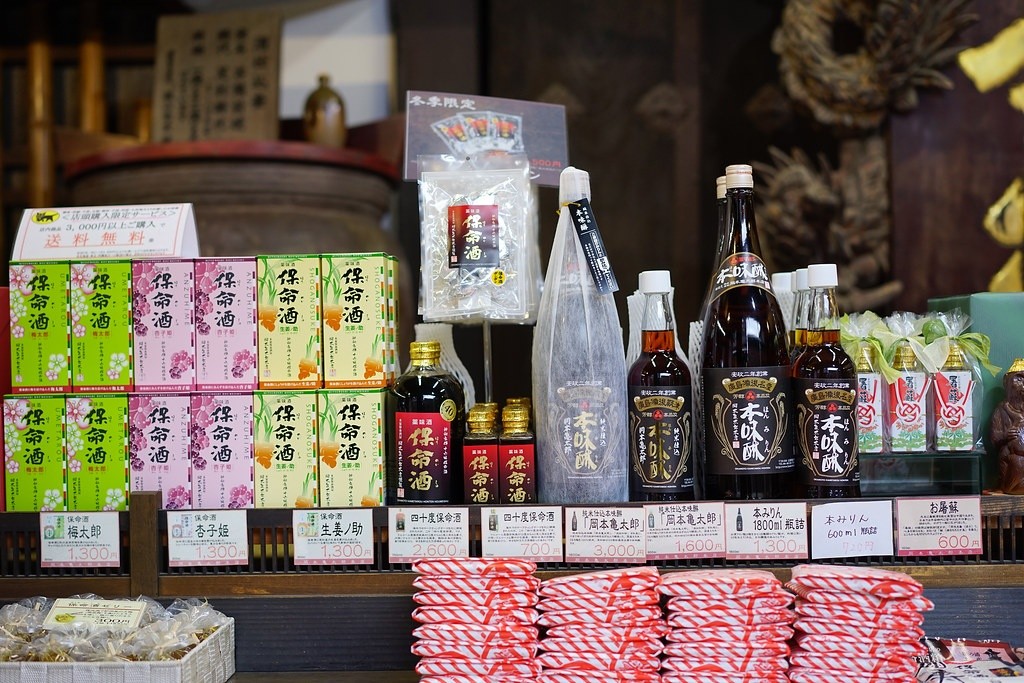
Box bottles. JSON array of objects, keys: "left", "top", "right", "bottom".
[
  {"left": 296, "top": 517, "right": 307, "bottom": 537},
  {"left": 736, "top": 508, "right": 743, "bottom": 532},
  {"left": 395, "top": 509, "right": 406, "bottom": 533},
  {"left": 171, "top": 519, "right": 183, "bottom": 539},
  {"left": 648, "top": 512, "right": 655, "bottom": 528},
  {"left": 489, "top": 509, "right": 499, "bottom": 531},
  {"left": 389, "top": 165, "right": 980, "bottom": 506},
  {"left": 44, "top": 520, "right": 55, "bottom": 540},
  {"left": 304, "top": 74, "right": 346, "bottom": 147},
  {"left": 572, "top": 511, "right": 577, "bottom": 531}
]
